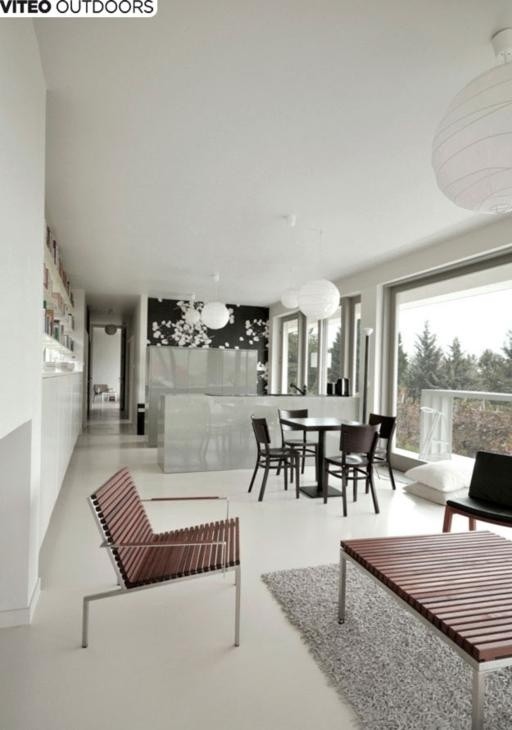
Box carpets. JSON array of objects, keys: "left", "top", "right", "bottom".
[{"left": 262, "top": 563, "right": 512, "bottom": 729}]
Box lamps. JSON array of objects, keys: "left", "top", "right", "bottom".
[
  {"left": 429, "top": 26, "right": 511, "bottom": 215},
  {"left": 199, "top": 231, "right": 341, "bottom": 331}
]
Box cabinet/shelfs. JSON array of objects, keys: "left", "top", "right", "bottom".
[{"left": 42, "top": 242, "right": 82, "bottom": 378}]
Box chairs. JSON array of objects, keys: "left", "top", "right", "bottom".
[
  {"left": 92, "top": 384, "right": 117, "bottom": 405},
  {"left": 82, "top": 467, "right": 242, "bottom": 646},
  {"left": 248, "top": 409, "right": 396, "bottom": 517},
  {"left": 441, "top": 452, "right": 511, "bottom": 533}
]
